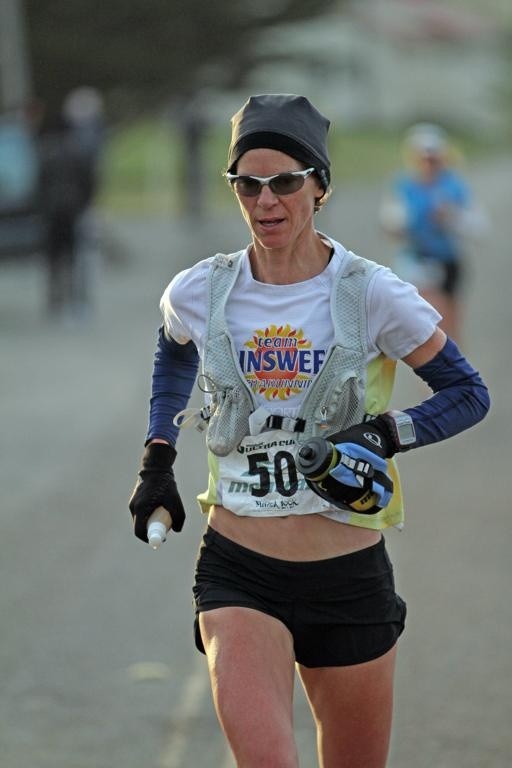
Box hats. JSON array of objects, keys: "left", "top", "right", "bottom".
[{"left": 226, "top": 94, "right": 330, "bottom": 202}]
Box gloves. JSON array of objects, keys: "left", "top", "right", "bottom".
[
  {"left": 304, "top": 414, "right": 399, "bottom": 499},
  {"left": 129, "top": 443, "right": 185, "bottom": 543}
]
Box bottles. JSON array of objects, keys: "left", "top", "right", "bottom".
[
  {"left": 292, "top": 439, "right": 338, "bottom": 481},
  {"left": 146, "top": 502, "right": 174, "bottom": 551}
]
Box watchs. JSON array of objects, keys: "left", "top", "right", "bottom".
[{"left": 378, "top": 409, "right": 416, "bottom": 447}]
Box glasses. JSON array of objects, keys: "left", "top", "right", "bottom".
[{"left": 226, "top": 167, "right": 314, "bottom": 197}]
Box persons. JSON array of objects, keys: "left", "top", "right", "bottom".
[
  {"left": 377, "top": 127, "right": 487, "bottom": 343},
  {"left": 127, "top": 93, "right": 491, "bottom": 768}
]
